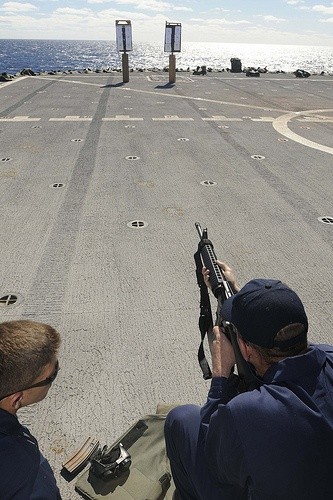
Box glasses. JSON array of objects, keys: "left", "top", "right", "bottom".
[{"left": 0, "top": 359, "right": 59, "bottom": 400}]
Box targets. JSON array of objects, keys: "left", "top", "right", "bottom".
[
  {"left": 115, "top": 19, "right": 133, "bottom": 52},
  {"left": 164, "top": 21, "right": 182, "bottom": 52}
]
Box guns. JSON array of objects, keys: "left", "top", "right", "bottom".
[{"left": 194, "top": 222, "right": 259, "bottom": 392}]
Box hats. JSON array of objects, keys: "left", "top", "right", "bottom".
[{"left": 221, "top": 279, "right": 308, "bottom": 348}]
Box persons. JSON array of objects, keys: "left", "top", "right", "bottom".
[
  {"left": 0, "top": 319, "right": 63, "bottom": 500},
  {"left": 163, "top": 260, "right": 333, "bottom": 500}
]
